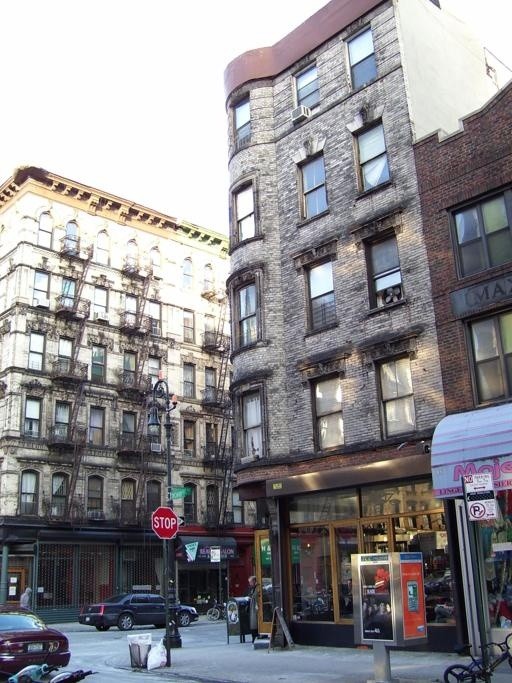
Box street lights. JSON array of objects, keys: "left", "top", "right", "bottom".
[{"left": 148, "top": 379, "right": 183, "bottom": 648}]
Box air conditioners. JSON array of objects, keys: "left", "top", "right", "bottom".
[
  {"left": 291, "top": 106, "right": 311, "bottom": 124},
  {"left": 150, "top": 443, "right": 162, "bottom": 453}
]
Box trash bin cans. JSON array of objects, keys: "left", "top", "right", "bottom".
[{"left": 127, "top": 633, "right": 152, "bottom": 669}]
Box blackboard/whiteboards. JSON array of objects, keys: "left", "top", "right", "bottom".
[{"left": 268, "top": 606, "right": 293, "bottom": 648}]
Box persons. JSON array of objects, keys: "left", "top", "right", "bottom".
[
  {"left": 248, "top": 575, "right": 258, "bottom": 642},
  {"left": 347, "top": 579, "right": 352, "bottom": 595},
  {"left": 20, "top": 587, "right": 32, "bottom": 610},
  {"left": 363, "top": 601, "right": 391, "bottom": 628}
]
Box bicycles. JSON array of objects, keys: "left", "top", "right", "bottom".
[
  {"left": 207, "top": 595, "right": 229, "bottom": 621},
  {"left": 444, "top": 633, "right": 511, "bottom": 683}
]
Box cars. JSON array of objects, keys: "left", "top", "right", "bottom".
[
  {"left": 0, "top": 603, "right": 70, "bottom": 674},
  {"left": 79, "top": 590, "right": 200, "bottom": 631}
]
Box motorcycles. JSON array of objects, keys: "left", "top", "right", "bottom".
[{"left": 0, "top": 651, "right": 97, "bottom": 683}]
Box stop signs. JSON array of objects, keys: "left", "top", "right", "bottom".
[{"left": 151, "top": 506, "right": 180, "bottom": 540}]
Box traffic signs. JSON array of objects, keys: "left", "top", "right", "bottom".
[
  {"left": 290, "top": 538, "right": 301, "bottom": 564},
  {"left": 252, "top": 538, "right": 272, "bottom": 565}
]
